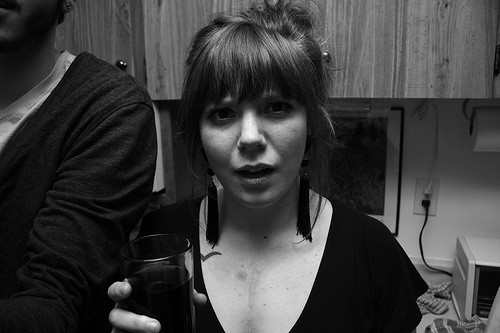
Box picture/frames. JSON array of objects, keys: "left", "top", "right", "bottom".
[{"left": 321, "top": 106, "right": 407, "bottom": 238}]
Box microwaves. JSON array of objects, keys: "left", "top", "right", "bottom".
[{"left": 450, "top": 236, "right": 500, "bottom": 320}]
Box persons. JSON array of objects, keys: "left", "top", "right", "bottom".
[
  {"left": 107, "top": 1, "right": 430, "bottom": 333},
  {"left": 0, "top": 0, "right": 158, "bottom": 333}
]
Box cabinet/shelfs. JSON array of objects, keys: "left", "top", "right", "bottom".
[{"left": 53, "top": 0, "right": 500, "bottom": 102}]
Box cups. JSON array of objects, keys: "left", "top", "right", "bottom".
[{"left": 122, "top": 234, "right": 195, "bottom": 333}]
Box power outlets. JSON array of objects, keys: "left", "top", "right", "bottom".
[{"left": 413, "top": 176, "right": 440, "bottom": 217}]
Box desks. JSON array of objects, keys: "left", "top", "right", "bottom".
[{"left": 412, "top": 263, "right": 500, "bottom": 333}]
